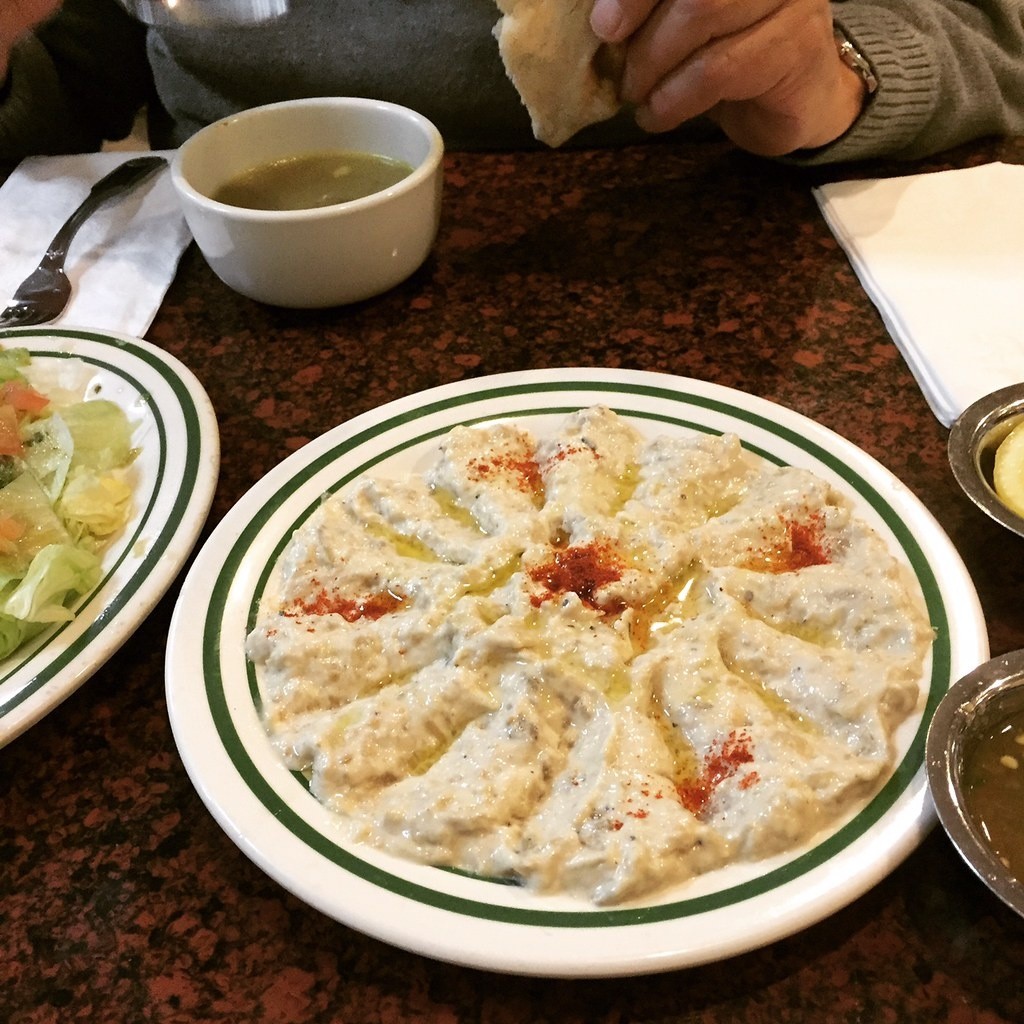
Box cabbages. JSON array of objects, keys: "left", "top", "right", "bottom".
[{"left": 0, "top": 348, "right": 140, "bottom": 661}]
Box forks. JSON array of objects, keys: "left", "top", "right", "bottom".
[{"left": 0, "top": 156, "right": 170, "bottom": 347}]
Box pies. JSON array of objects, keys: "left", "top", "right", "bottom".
[{"left": 492, "top": 0, "right": 627, "bottom": 146}]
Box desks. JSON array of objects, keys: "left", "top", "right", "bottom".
[{"left": 0, "top": 137, "right": 1024, "bottom": 1024}]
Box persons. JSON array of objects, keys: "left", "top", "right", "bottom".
[{"left": 0, "top": 0, "right": 1023, "bottom": 170}]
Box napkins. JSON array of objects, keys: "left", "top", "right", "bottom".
[
  {"left": 810, "top": 159, "right": 1024, "bottom": 430},
  {"left": 0, "top": 148, "right": 194, "bottom": 340}
]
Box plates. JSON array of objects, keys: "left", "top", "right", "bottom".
[
  {"left": 0, "top": 326, "right": 221, "bottom": 752},
  {"left": 163, "top": 365, "right": 990, "bottom": 979}
]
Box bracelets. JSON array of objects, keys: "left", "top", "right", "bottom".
[{"left": 833, "top": 26, "right": 878, "bottom": 93}]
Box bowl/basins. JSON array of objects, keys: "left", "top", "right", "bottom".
[
  {"left": 946, "top": 383, "right": 1024, "bottom": 539},
  {"left": 926, "top": 647, "right": 1024, "bottom": 919},
  {"left": 170, "top": 96, "right": 445, "bottom": 310}
]
